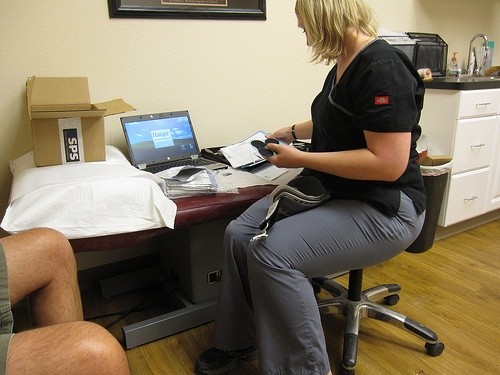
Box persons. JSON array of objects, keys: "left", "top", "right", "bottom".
[
  {"left": 1, "top": 227, "right": 130, "bottom": 374},
  {"left": 191, "top": 0, "right": 428, "bottom": 375}
]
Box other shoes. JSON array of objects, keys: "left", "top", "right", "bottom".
[{"left": 195, "top": 342, "right": 258, "bottom": 375}]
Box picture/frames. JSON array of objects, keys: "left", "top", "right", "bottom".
[{"left": 107, "top": 0, "right": 267, "bottom": 21}]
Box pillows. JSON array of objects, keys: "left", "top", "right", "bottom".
[{"left": 0, "top": 144, "right": 178, "bottom": 241}]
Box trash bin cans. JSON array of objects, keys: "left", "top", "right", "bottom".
[{"left": 404, "top": 158, "right": 453, "bottom": 253}]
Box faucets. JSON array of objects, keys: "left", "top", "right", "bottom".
[{"left": 465, "top": 33, "right": 488, "bottom": 75}]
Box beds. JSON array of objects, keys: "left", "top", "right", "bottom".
[{"left": 66, "top": 184, "right": 279, "bottom": 350}]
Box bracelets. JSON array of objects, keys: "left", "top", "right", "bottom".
[{"left": 290, "top": 125, "right": 297, "bottom": 140}]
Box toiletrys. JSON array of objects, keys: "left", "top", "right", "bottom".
[{"left": 448, "top": 52, "right": 459, "bottom": 76}]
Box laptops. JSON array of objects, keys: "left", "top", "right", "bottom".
[{"left": 119, "top": 111, "right": 228, "bottom": 176}]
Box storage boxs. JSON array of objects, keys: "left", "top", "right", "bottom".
[{"left": 25, "top": 74, "right": 137, "bottom": 167}]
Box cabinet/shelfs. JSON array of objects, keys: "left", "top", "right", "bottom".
[{"left": 415, "top": 88, "right": 500, "bottom": 242}]
